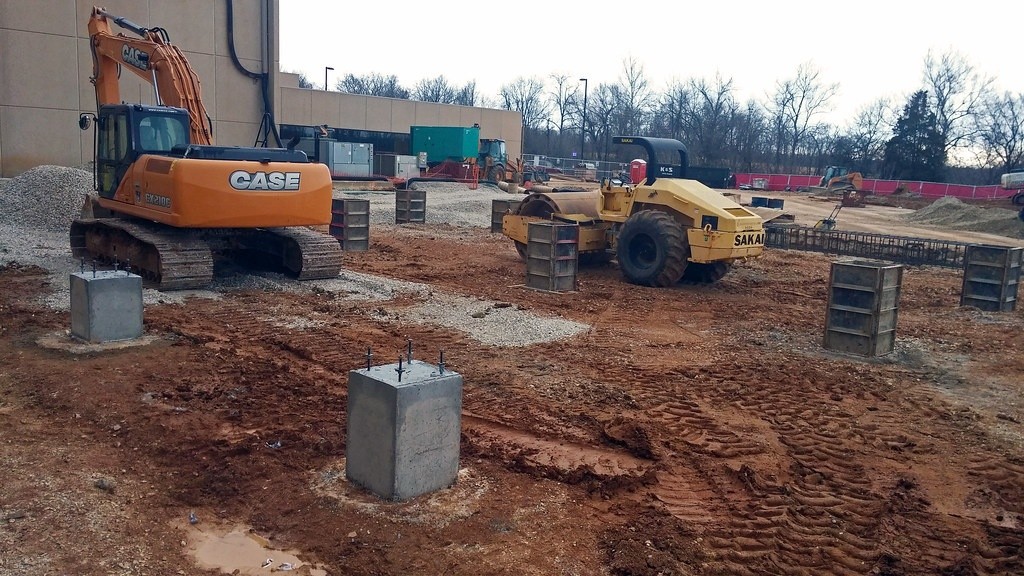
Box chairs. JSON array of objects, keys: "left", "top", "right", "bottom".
[{"left": 140, "top": 126, "right": 158, "bottom": 150}]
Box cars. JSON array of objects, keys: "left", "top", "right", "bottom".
[
  {"left": 578, "top": 160, "right": 590, "bottom": 167},
  {"left": 524, "top": 160, "right": 565, "bottom": 175},
  {"left": 523, "top": 164, "right": 550, "bottom": 181}
]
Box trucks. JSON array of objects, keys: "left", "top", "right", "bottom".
[{"left": 410, "top": 125, "right": 508, "bottom": 184}]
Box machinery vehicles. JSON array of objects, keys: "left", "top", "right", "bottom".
[
  {"left": 491, "top": 136, "right": 766, "bottom": 287},
  {"left": 819, "top": 165, "right": 862, "bottom": 190},
  {"left": 1000, "top": 171, "right": 1024, "bottom": 205},
  {"left": 70, "top": 6, "right": 344, "bottom": 291}
]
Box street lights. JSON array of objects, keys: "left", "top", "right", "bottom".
[
  {"left": 602, "top": 124, "right": 608, "bottom": 176},
  {"left": 580, "top": 79, "right": 587, "bottom": 159},
  {"left": 325, "top": 67, "right": 334, "bottom": 90}
]
